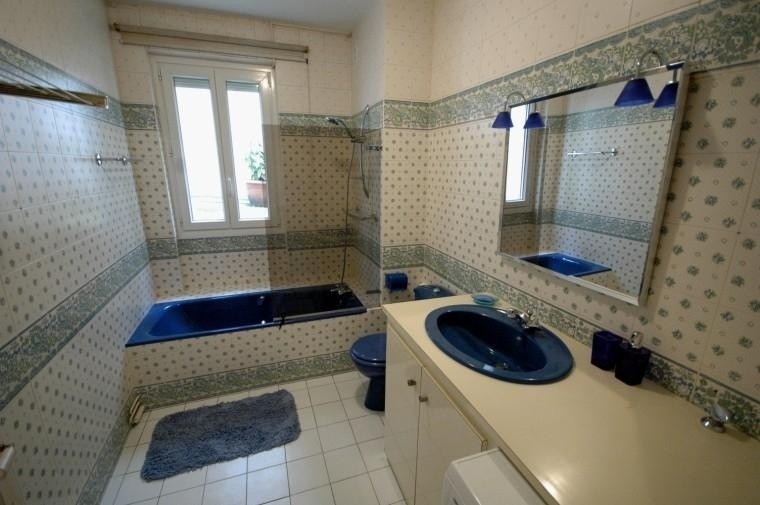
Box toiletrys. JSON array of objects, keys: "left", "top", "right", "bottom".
[{"left": 614, "top": 332, "right": 651, "bottom": 386}]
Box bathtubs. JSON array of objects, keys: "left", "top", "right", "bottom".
[{"left": 519, "top": 252, "right": 613, "bottom": 277}]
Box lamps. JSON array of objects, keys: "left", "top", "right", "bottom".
[
  {"left": 611, "top": 45, "right": 663, "bottom": 110},
  {"left": 523, "top": 103, "right": 546, "bottom": 130},
  {"left": 492, "top": 86, "right": 528, "bottom": 131},
  {"left": 652, "top": 68, "right": 679, "bottom": 110}
]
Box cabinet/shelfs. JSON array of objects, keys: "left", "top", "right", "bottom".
[{"left": 382, "top": 322, "right": 488, "bottom": 505}]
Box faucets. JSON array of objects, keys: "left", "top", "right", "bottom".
[
  {"left": 507, "top": 310, "right": 542, "bottom": 333},
  {"left": 330, "top": 283, "right": 352, "bottom": 294}
]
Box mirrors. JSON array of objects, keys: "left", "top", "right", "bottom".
[{"left": 495, "top": 56, "right": 693, "bottom": 306}]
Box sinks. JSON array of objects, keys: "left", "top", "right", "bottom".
[
  {"left": 125, "top": 283, "right": 366, "bottom": 348},
  {"left": 426, "top": 304, "right": 574, "bottom": 384}
]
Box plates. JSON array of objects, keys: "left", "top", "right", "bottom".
[{"left": 471, "top": 293, "right": 501, "bottom": 306}]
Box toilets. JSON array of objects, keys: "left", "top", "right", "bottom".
[{"left": 350, "top": 283, "right": 456, "bottom": 411}]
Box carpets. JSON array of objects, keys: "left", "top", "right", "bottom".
[{"left": 136, "top": 387, "right": 302, "bottom": 484}]
